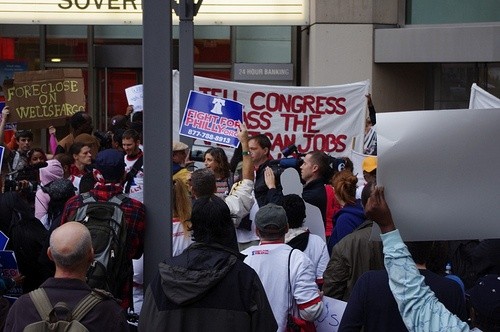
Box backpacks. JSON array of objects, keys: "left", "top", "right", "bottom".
[
  {"left": 0, "top": 191, "right": 56, "bottom": 298},
  {"left": 70, "top": 192, "right": 130, "bottom": 303},
  {"left": 22, "top": 287, "right": 115, "bottom": 332}
]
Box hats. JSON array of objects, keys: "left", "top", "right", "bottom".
[
  {"left": 94, "top": 148, "right": 127, "bottom": 173},
  {"left": 362, "top": 156, "right": 377, "bottom": 173},
  {"left": 254, "top": 203, "right": 289, "bottom": 234},
  {"left": 173, "top": 140, "right": 189, "bottom": 152},
  {"left": 49, "top": 178, "right": 78, "bottom": 200}
]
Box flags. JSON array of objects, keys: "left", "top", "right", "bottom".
[{"left": 172, "top": 69, "right": 370, "bottom": 188}]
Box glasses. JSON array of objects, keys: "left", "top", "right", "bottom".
[{"left": 17, "top": 128, "right": 32, "bottom": 133}]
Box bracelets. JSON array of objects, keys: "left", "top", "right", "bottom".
[{"left": 242, "top": 151, "right": 250, "bottom": 155}]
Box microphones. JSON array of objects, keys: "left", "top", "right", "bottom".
[{"left": 8, "top": 162, "right": 48, "bottom": 176}]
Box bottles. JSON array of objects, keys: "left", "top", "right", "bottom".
[{"left": 444, "top": 266, "right": 452, "bottom": 275}]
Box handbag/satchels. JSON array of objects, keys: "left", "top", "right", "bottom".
[{"left": 285, "top": 314, "right": 317, "bottom": 332}]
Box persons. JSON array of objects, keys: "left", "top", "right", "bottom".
[
  {"left": 364, "top": 185, "right": 500, "bottom": 332},
  {"left": 237, "top": 202, "right": 326, "bottom": 332},
  {"left": 139, "top": 196, "right": 277, "bottom": 330},
  {"left": 4, "top": 221, "right": 128, "bottom": 332},
  {"left": 1, "top": 94, "right": 500, "bottom": 318}
]
